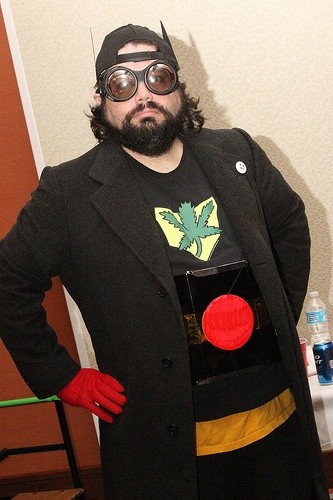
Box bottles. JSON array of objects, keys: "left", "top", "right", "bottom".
[{"left": 306, "top": 292, "right": 330, "bottom": 343}]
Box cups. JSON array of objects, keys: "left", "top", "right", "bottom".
[{"left": 300, "top": 339, "right": 308, "bottom": 375}]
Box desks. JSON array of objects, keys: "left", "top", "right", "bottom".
[{"left": 299, "top": 346, "right": 333, "bottom": 452}]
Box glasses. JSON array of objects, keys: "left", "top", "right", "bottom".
[{"left": 98, "top": 59, "right": 178, "bottom": 102}]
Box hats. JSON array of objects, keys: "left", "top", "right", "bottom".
[{"left": 95, "top": 24, "right": 180, "bottom": 83}]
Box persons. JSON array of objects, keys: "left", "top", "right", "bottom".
[{"left": 0, "top": 24, "right": 330, "bottom": 500}]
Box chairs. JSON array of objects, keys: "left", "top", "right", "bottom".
[{"left": 0, "top": 395, "right": 84, "bottom": 500}]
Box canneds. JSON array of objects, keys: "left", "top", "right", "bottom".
[{"left": 313, "top": 340, "right": 333, "bottom": 386}]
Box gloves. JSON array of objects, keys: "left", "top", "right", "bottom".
[{"left": 55, "top": 367, "right": 127, "bottom": 424}]
